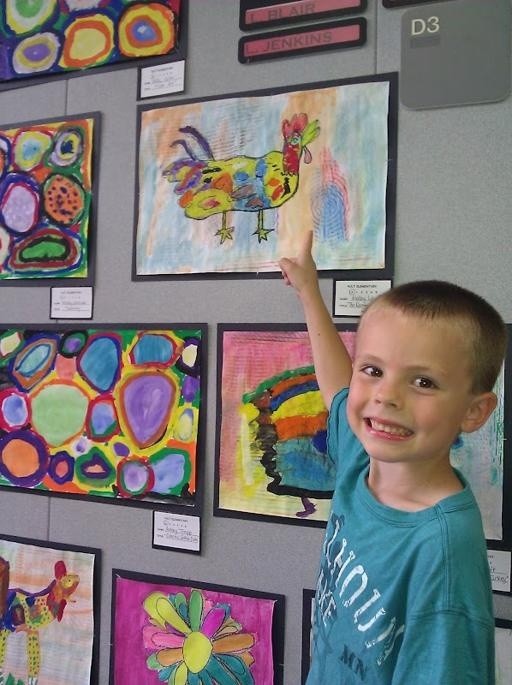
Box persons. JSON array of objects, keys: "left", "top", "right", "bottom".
[{"left": 279, "top": 231, "right": 507, "bottom": 685}]
